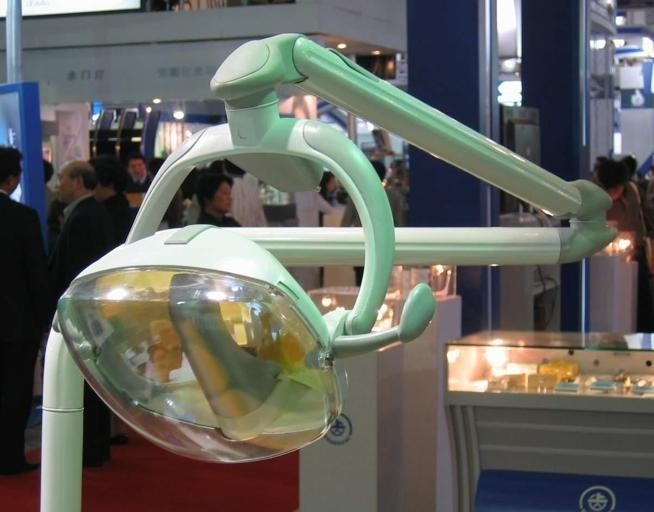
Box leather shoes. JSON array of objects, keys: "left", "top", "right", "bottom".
[
  {"left": 110, "top": 433, "right": 129, "bottom": 445},
  {"left": 0, "top": 463, "right": 40, "bottom": 475}
]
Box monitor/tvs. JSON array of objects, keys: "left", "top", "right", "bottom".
[{"left": 500, "top": 106, "right": 543, "bottom": 212}]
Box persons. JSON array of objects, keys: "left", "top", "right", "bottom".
[
  {"left": 318, "top": 126, "right": 409, "bottom": 287},
  {"left": 149, "top": 154, "right": 270, "bottom": 229},
  {"left": 588, "top": 152, "right": 654, "bottom": 332}
]
